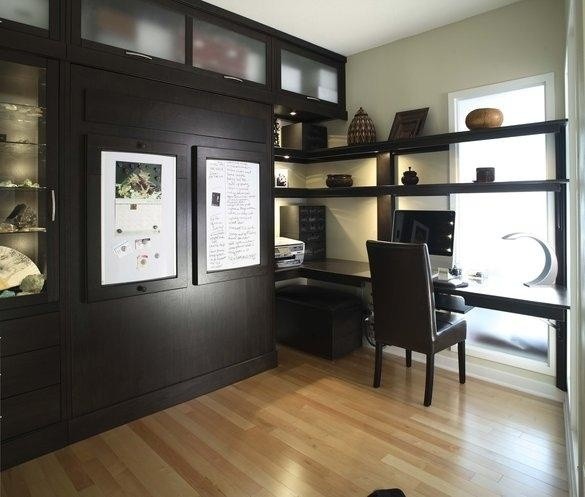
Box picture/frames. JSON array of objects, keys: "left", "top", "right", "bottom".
[{"left": 80, "top": 132, "right": 269, "bottom": 304}]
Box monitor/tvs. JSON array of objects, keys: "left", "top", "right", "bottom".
[{"left": 391, "top": 209, "right": 456, "bottom": 279}]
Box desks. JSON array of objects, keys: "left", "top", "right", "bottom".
[{"left": 275, "top": 257, "right": 570, "bottom": 394}]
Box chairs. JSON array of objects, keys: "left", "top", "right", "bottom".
[{"left": 365, "top": 238, "right": 468, "bottom": 406}]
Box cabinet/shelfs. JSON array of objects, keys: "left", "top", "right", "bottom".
[
  {"left": 0, "top": 0, "right": 348, "bottom": 121},
  {"left": 274, "top": 117, "right": 571, "bottom": 289},
  {"left": 0, "top": 44, "right": 63, "bottom": 470}
]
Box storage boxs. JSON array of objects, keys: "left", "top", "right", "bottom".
[{"left": 281, "top": 121, "right": 328, "bottom": 151}]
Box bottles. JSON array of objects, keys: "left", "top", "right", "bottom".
[{"left": 401, "top": 167, "right": 419, "bottom": 186}]
